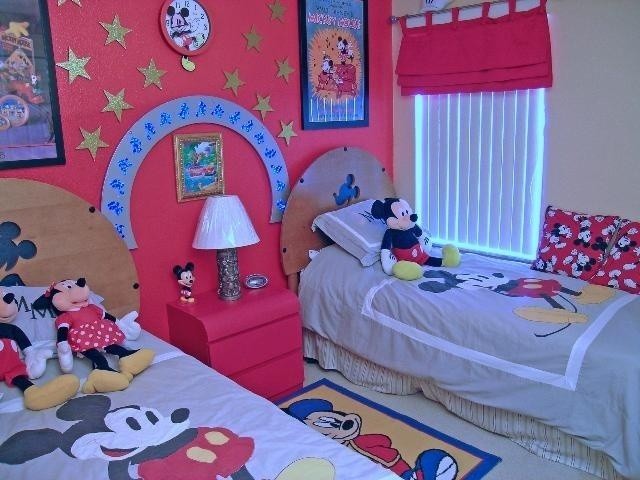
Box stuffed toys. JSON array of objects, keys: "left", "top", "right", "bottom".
[
  {"left": 0, "top": 289, "right": 81, "bottom": 411},
  {"left": 172, "top": 262, "right": 195, "bottom": 304},
  {"left": 371, "top": 197, "right": 460, "bottom": 281},
  {"left": 31, "top": 278, "right": 154, "bottom": 393}
]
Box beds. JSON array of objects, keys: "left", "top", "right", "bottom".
[
  {"left": 0, "top": 175, "right": 403, "bottom": 480},
  {"left": 278, "top": 146, "right": 639, "bottom": 480}
]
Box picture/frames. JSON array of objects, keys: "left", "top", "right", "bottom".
[
  {"left": 0, "top": 1, "right": 69, "bottom": 173},
  {"left": 298, "top": 0, "right": 370, "bottom": 131},
  {"left": 172, "top": 131, "right": 226, "bottom": 204}
]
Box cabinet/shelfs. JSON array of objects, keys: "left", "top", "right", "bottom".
[{"left": 165, "top": 281, "right": 305, "bottom": 400}]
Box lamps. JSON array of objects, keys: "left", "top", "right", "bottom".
[{"left": 192, "top": 193, "right": 261, "bottom": 300}]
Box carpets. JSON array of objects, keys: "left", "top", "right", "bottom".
[{"left": 274, "top": 377, "right": 503, "bottom": 480}]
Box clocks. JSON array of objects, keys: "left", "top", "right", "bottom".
[{"left": 159, "top": 0, "right": 213, "bottom": 58}]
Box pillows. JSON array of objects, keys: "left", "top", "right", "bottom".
[
  {"left": 528, "top": 203, "right": 640, "bottom": 296},
  {"left": 0, "top": 284, "right": 104, "bottom": 362},
  {"left": 311, "top": 198, "right": 387, "bottom": 268}
]
